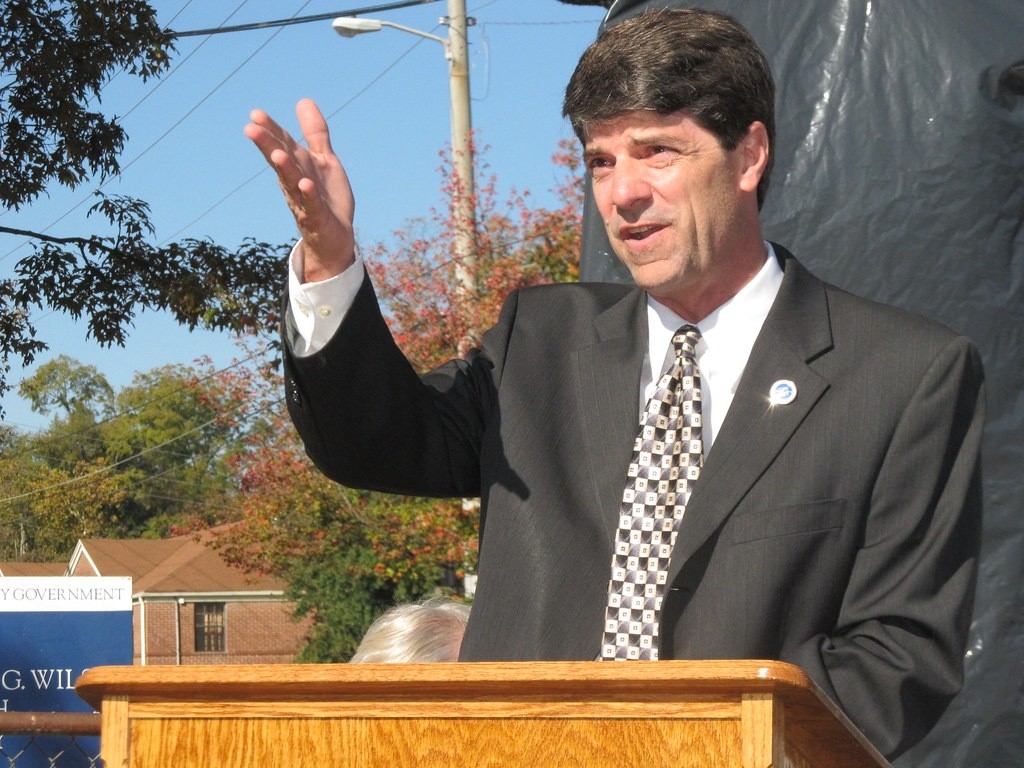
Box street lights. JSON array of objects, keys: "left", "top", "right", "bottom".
[{"left": 332, "top": 17, "right": 477, "bottom": 597}]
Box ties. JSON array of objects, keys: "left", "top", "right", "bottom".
[{"left": 599, "top": 324, "right": 703, "bottom": 662}]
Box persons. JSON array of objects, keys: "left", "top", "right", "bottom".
[
  {"left": 344, "top": 592, "right": 476, "bottom": 664},
  {"left": 244, "top": 5, "right": 985, "bottom": 762}
]
